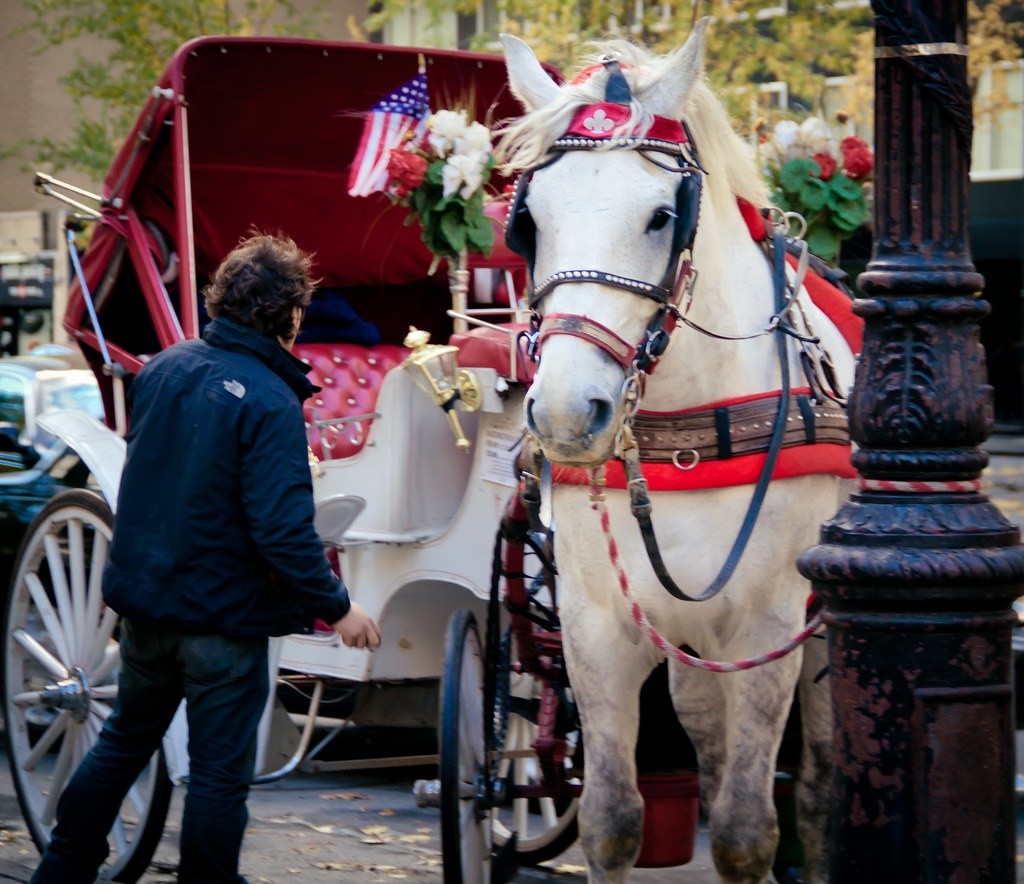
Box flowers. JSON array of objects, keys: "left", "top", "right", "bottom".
[
  {"left": 752, "top": 107, "right": 876, "bottom": 259},
  {"left": 382, "top": 108, "right": 500, "bottom": 276}
]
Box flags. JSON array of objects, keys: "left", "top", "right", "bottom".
[{"left": 347, "top": 73, "right": 435, "bottom": 206}]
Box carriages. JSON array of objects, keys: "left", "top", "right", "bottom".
[{"left": 2, "top": 34, "right": 863, "bottom": 884}]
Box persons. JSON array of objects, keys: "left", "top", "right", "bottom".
[{"left": 25, "top": 239, "right": 384, "bottom": 884}]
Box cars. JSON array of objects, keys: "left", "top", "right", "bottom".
[
  {"left": 5, "top": 346, "right": 121, "bottom": 590},
  {"left": 0, "top": 251, "right": 55, "bottom": 331}
]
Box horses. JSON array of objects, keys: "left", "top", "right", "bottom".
[{"left": 486, "top": 16, "right": 868, "bottom": 883}]
